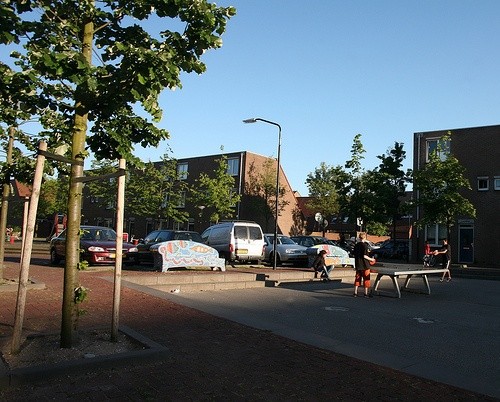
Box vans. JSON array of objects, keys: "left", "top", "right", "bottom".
[{"left": 202, "top": 219, "right": 266, "bottom": 264}]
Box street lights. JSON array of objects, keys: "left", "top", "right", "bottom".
[{"left": 242, "top": 117, "right": 282, "bottom": 271}]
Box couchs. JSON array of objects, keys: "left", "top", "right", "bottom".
[
  {"left": 306, "top": 244, "right": 355, "bottom": 268},
  {"left": 150, "top": 240, "right": 225, "bottom": 272}
]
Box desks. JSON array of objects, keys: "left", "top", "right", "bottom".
[{"left": 369, "top": 265, "right": 449, "bottom": 299}]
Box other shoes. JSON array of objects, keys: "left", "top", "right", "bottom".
[
  {"left": 446, "top": 278, "right": 451, "bottom": 283},
  {"left": 353, "top": 293, "right": 357, "bottom": 297},
  {"left": 364, "top": 294, "right": 367, "bottom": 296},
  {"left": 439, "top": 278, "right": 443, "bottom": 282}
]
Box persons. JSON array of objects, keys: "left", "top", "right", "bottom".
[
  {"left": 352, "top": 232, "right": 375, "bottom": 297},
  {"left": 423, "top": 238, "right": 452, "bottom": 283},
  {"left": 313, "top": 249, "right": 334, "bottom": 279}
]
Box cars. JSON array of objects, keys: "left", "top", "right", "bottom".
[
  {"left": 260, "top": 234, "right": 311, "bottom": 267},
  {"left": 49, "top": 225, "right": 139, "bottom": 268},
  {"left": 136, "top": 229, "right": 203, "bottom": 266},
  {"left": 290, "top": 235, "right": 409, "bottom": 262}
]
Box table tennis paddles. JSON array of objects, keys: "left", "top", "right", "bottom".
[{"left": 370, "top": 256, "right": 376, "bottom": 265}]
